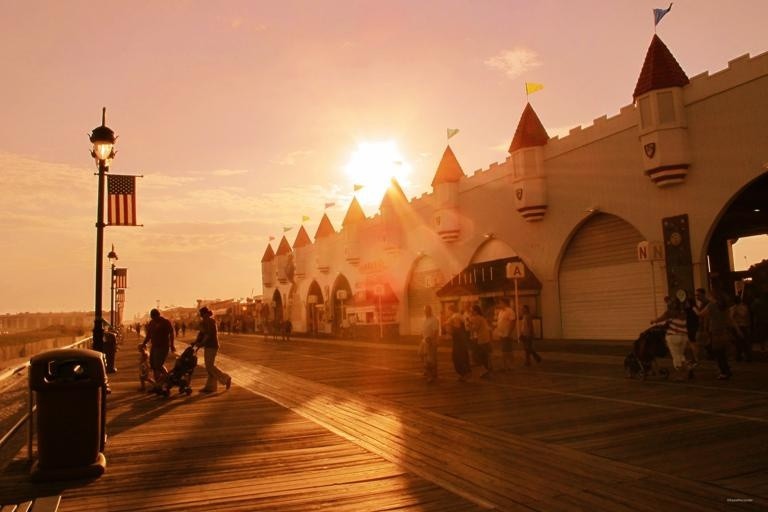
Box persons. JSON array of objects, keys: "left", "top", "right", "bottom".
[
  {"left": 263, "top": 317, "right": 292, "bottom": 341},
  {"left": 420, "top": 298, "right": 541, "bottom": 382},
  {"left": 652, "top": 290, "right": 750, "bottom": 377},
  {"left": 339, "top": 316, "right": 355, "bottom": 337},
  {"left": 145, "top": 309, "right": 177, "bottom": 379},
  {"left": 136, "top": 343, "right": 154, "bottom": 391},
  {"left": 192, "top": 308, "right": 231, "bottom": 393},
  {"left": 128, "top": 322, "right": 241, "bottom": 338}
]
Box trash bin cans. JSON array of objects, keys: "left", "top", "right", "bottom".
[{"left": 29, "top": 348, "right": 112, "bottom": 482}]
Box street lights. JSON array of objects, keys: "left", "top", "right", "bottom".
[
  {"left": 87, "top": 106, "right": 119, "bottom": 372},
  {"left": 107, "top": 243, "right": 127, "bottom": 351}
]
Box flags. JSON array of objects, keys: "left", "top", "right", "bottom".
[
  {"left": 108, "top": 174, "right": 135, "bottom": 225},
  {"left": 652, "top": 2, "right": 672, "bottom": 25},
  {"left": 526, "top": 83, "right": 545, "bottom": 94},
  {"left": 267, "top": 160, "right": 402, "bottom": 242},
  {"left": 447, "top": 128, "right": 457, "bottom": 138},
  {"left": 118, "top": 290, "right": 125, "bottom": 301},
  {"left": 115, "top": 268, "right": 126, "bottom": 288}
]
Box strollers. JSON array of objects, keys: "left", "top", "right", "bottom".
[
  {"left": 622, "top": 321, "right": 671, "bottom": 382},
  {"left": 148, "top": 342, "right": 205, "bottom": 398}
]
